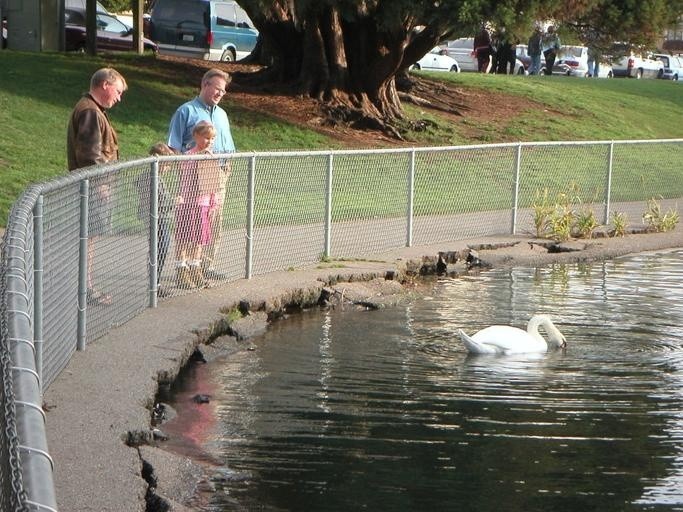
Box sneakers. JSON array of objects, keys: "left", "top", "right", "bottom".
[{"left": 148, "top": 265, "right": 225, "bottom": 298}]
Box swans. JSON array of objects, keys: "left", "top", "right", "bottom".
[{"left": 456, "top": 313, "right": 568, "bottom": 355}]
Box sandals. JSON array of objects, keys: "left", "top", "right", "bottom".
[{"left": 86, "top": 287, "right": 115, "bottom": 307}]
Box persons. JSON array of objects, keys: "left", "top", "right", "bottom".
[
  {"left": 585, "top": 26, "right": 607, "bottom": 79},
  {"left": 133, "top": 142, "right": 182, "bottom": 300},
  {"left": 175, "top": 122, "right": 217, "bottom": 290},
  {"left": 473, "top": 18, "right": 517, "bottom": 75},
  {"left": 170, "top": 68, "right": 235, "bottom": 283},
  {"left": 526, "top": 25, "right": 545, "bottom": 77},
  {"left": 543, "top": 24, "right": 564, "bottom": 77},
  {"left": 67, "top": 66, "right": 127, "bottom": 309}
]
